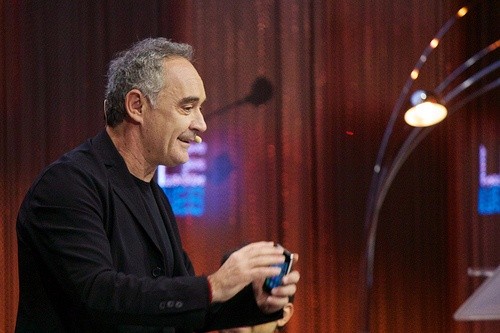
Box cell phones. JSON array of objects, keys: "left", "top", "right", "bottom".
[{"left": 263, "top": 247, "right": 293, "bottom": 296}]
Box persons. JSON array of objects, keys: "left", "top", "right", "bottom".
[
  {"left": 15, "top": 37, "right": 302, "bottom": 333},
  {"left": 217, "top": 247, "right": 294, "bottom": 333}
]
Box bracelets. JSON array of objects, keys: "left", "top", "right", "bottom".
[{"left": 207, "top": 279, "right": 212, "bottom": 303}]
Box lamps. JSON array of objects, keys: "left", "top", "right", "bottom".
[{"left": 404, "top": 87, "right": 449, "bottom": 129}]
